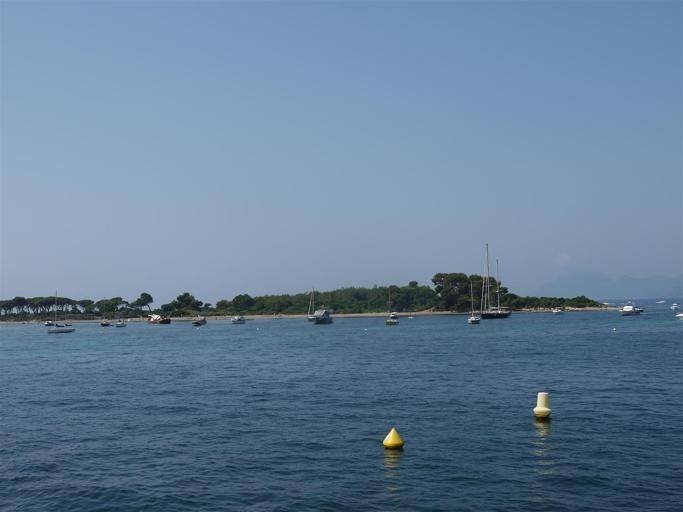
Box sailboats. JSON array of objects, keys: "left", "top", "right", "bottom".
[
  {"left": 307, "top": 287, "right": 325, "bottom": 322},
  {"left": 479, "top": 243, "right": 511, "bottom": 319},
  {"left": 467, "top": 280, "right": 480, "bottom": 325}
]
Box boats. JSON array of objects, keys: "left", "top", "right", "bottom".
[
  {"left": 314, "top": 313, "right": 333, "bottom": 325},
  {"left": 655, "top": 300, "right": 683, "bottom": 319},
  {"left": 552, "top": 308, "right": 561, "bottom": 314},
  {"left": 231, "top": 316, "right": 246, "bottom": 324},
  {"left": 618, "top": 305, "right": 640, "bottom": 316},
  {"left": 116, "top": 320, "right": 127, "bottom": 327},
  {"left": 101, "top": 321, "right": 114, "bottom": 326},
  {"left": 45, "top": 320, "right": 75, "bottom": 333},
  {"left": 386, "top": 319, "right": 400, "bottom": 325},
  {"left": 390, "top": 311, "right": 401, "bottom": 319},
  {"left": 148, "top": 314, "right": 172, "bottom": 324},
  {"left": 192, "top": 314, "right": 207, "bottom": 326}
]
